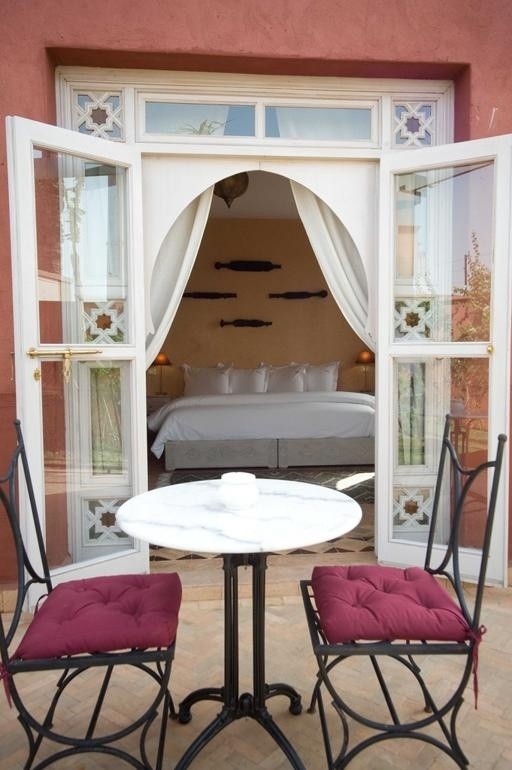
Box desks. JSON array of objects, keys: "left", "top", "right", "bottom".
[{"left": 114, "top": 479, "right": 364, "bottom": 770}]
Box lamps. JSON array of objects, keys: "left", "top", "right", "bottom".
[
  {"left": 153, "top": 353, "right": 172, "bottom": 394},
  {"left": 355, "top": 350, "right": 375, "bottom": 392}
]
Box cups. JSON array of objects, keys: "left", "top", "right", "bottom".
[
  {"left": 218, "top": 472, "right": 259, "bottom": 511},
  {"left": 450, "top": 399, "right": 467, "bottom": 414}
]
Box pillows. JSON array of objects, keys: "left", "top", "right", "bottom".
[
  {"left": 311, "top": 565, "right": 472, "bottom": 643},
  {"left": 260, "top": 362, "right": 309, "bottom": 392},
  {"left": 15, "top": 572, "right": 181, "bottom": 659},
  {"left": 290, "top": 361, "right": 340, "bottom": 392},
  {"left": 217, "top": 363, "right": 272, "bottom": 393},
  {"left": 182, "top": 363, "right": 233, "bottom": 396}
]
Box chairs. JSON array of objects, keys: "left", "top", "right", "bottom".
[
  {"left": 299, "top": 413, "right": 506, "bottom": 769},
  {"left": 0, "top": 418, "right": 183, "bottom": 770}
]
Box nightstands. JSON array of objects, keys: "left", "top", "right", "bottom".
[
  {"left": 363, "top": 392, "right": 375, "bottom": 396},
  {"left": 147, "top": 394, "right": 172, "bottom": 415}
]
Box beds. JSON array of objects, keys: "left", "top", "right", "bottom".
[{"left": 146, "top": 360, "right": 377, "bottom": 471}]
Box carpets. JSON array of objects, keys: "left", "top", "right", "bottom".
[{"left": 155, "top": 465, "right": 375, "bottom": 504}]
